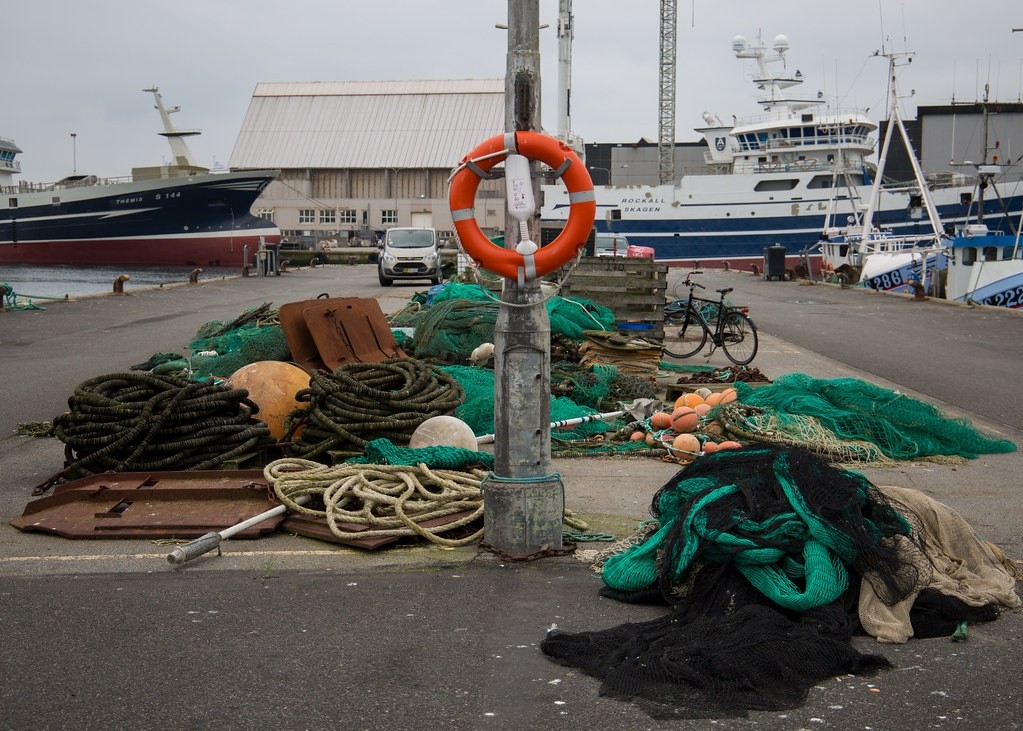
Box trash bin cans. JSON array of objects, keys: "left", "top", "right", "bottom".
[
  {"left": 262, "top": 242, "right": 278, "bottom": 274},
  {"left": 763, "top": 246, "right": 786, "bottom": 281}
]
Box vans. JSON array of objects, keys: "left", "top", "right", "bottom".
[{"left": 376, "top": 228, "right": 443, "bottom": 285}]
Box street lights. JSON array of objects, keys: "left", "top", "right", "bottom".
[{"left": 70, "top": 134, "right": 76, "bottom": 174}]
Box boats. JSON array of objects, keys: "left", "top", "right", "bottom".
[
  {"left": 821, "top": 43, "right": 1022, "bottom": 311},
  {"left": 538, "top": 34, "right": 1022, "bottom": 281},
  {"left": 0, "top": 88, "right": 283, "bottom": 269}
]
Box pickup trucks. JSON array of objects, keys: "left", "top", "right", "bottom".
[{"left": 438, "top": 248, "right": 458, "bottom": 279}]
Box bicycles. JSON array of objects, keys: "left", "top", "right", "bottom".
[{"left": 662, "top": 271, "right": 760, "bottom": 365}]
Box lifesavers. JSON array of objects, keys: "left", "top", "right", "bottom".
[{"left": 446, "top": 130, "right": 598, "bottom": 282}]
